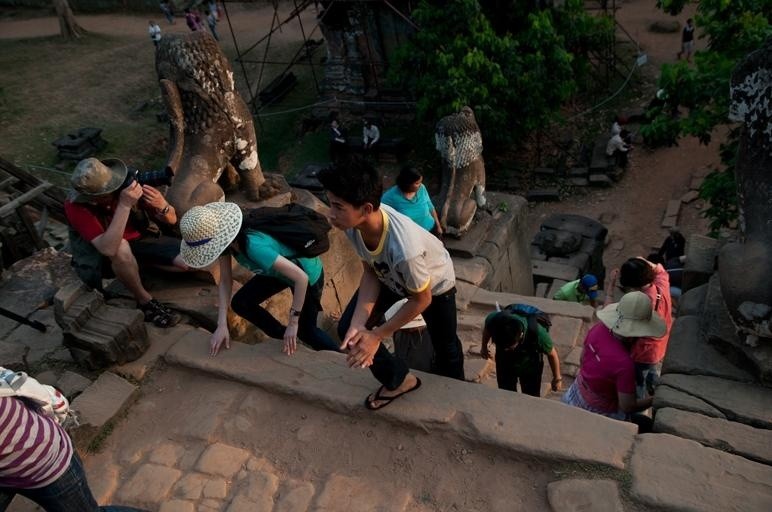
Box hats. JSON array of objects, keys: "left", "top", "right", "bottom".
[
  {"left": 596, "top": 291, "right": 668, "bottom": 339},
  {"left": 180, "top": 200, "right": 244, "bottom": 269},
  {"left": 71, "top": 157, "right": 128, "bottom": 197},
  {"left": 581, "top": 275, "right": 598, "bottom": 298},
  {"left": 385, "top": 298, "right": 427, "bottom": 329}
]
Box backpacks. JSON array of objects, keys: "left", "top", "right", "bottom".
[
  {"left": 504, "top": 303, "right": 551, "bottom": 331},
  {"left": 244, "top": 203, "right": 330, "bottom": 259},
  {"left": 1, "top": 366, "right": 70, "bottom": 425}
]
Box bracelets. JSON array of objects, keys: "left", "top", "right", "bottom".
[{"left": 552, "top": 378, "right": 563, "bottom": 385}]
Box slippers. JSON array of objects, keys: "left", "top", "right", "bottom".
[{"left": 365, "top": 376, "right": 420, "bottom": 412}]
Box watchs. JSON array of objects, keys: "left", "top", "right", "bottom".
[
  {"left": 159, "top": 201, "right": 173, "bottom": 217},
  {"left": 289, "top": 306, "right": 304, "bottom": 320}
]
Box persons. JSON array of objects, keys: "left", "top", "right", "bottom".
[
  {"left": 148, "top": 0, "right": 220, "bottom": 47},
  {"left": 65, "top": 158, "right": 196, "bottom": 329},
  {"left": 362, "top": 121, "right": 380, "bottom": 162},
  {"left": 317, "top": 158, "right": 464, "bottom": 410},
  {"left": 328, "top": 120, "right": 347, "bottom": 165},
  {"left": 475, "top": 300, "right": 563, "bottom": 399},
  {"left": 600, "top": 256, "right": 677, "bottom": 408},
  {"left": 605, "top": 85, "right": 679, "bottom": 167},
  {"left": 178, "top": 198, "right": 337, "bottom": 356},
  {"left": 676, "top": 17, "right": 699, "bottom": 59},
  {"left": 643, "top": 226, "right": 687, "bottom": 276},
  {"left": 0, "top": 362, "right": 141, "bottom": 511},
  {"left": 553, "top": 274, "right": 599, "bottom": 316},
  {"left": 376, "top": 164, "right": 445, "bottom": 248},
  {"left": 561, "top": 292, "right": 668, "bottom": 428}
]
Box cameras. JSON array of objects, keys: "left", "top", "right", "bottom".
[{"left": 114, "top": 165, "right": 175, "bottom": 201}]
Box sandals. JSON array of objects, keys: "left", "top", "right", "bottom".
[{"left": 137, "top": 296, "right": 182, "bottom": 327}]
